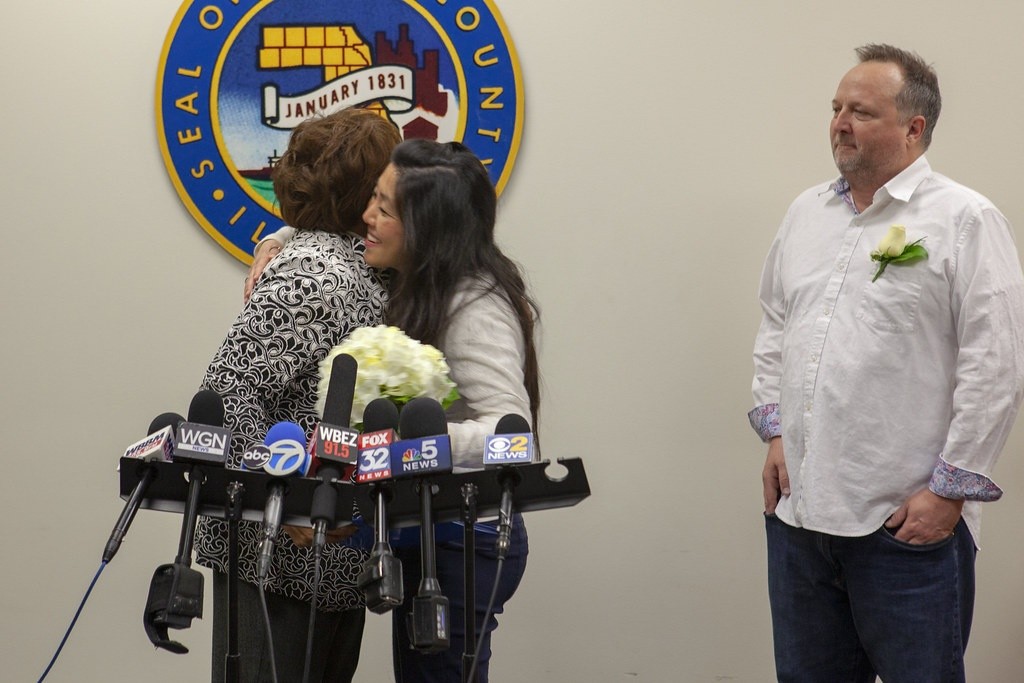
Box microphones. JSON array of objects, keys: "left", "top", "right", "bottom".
[{"left": 104, "top": 354, "right": 539, "bottom": 656}]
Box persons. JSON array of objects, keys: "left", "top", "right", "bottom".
[
  {"left": 242, "top": 137, "right": 540, "bottom": 683},
  {"left": 746, "top": 43, "right": 1023, "bottom": 683},
  {"left": 187, "top": 105, "right": 412, "bottom": 683}
]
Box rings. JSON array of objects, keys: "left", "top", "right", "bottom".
[
  {"left": 244, "top": 276, "right": 250, "bottom": 282},
  {"left": 268, "top": 243, "right": 282, "bottom": 253}
]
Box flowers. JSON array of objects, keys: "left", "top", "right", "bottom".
[
  {"left": 312, "top": 324, "right": 463, "bottom": 440},
  {"left": 868, "top": 224, "right": 929, "bottom": 284}
]
